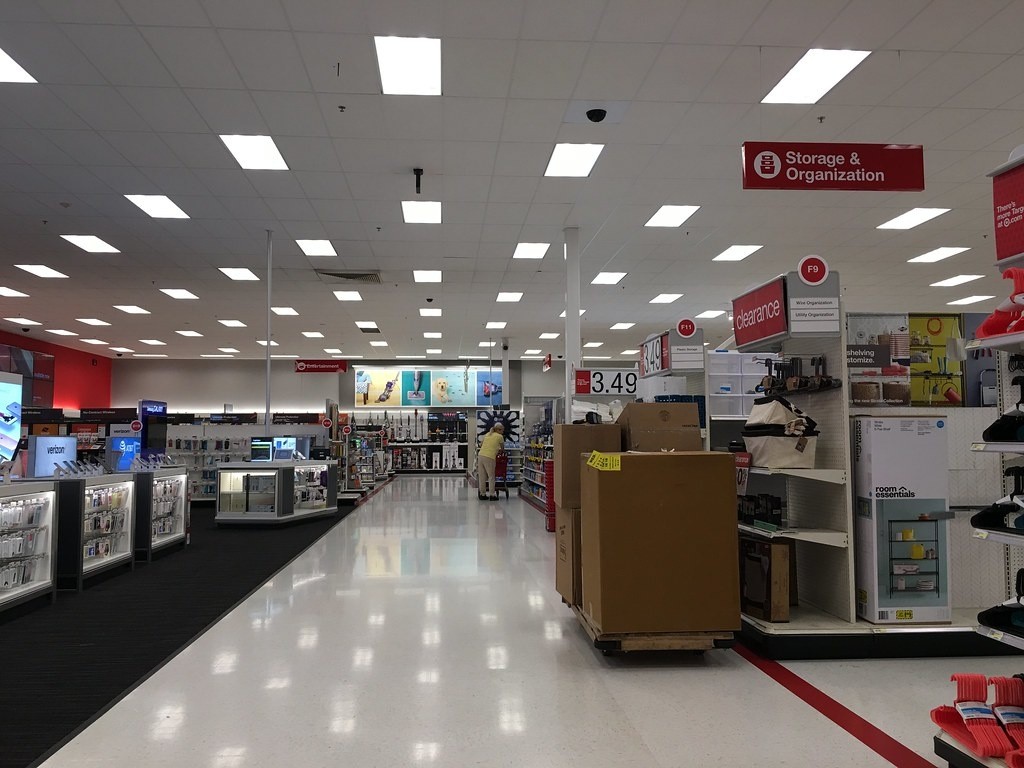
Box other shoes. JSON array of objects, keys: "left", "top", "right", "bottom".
[
  {"left": 490, "top": 495, "right": 499, "bottom": 501},
  {"left": 480, "top": 494, "right": 489, "bottom": 500}
]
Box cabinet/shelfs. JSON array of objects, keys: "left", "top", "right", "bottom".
[
  {"left": 932, "top": 255, "right": 1024, "bottom": 768},
  {"left": 351, "top": 436, "right": 377, "bottom": 490},
  {"left": 356, "top": 431, "right": 388, "bottom": 482},
  {"left": 737, "top": 301, "right": 874, "bottom": 661},
  {"left": 707, "top": 349, "right": 778, "bottom": 421},
  {"left": 388, "top": 442, "right": 467, "bottom": 474},
  {"left": 166, "top": 452, "right": 250, "bottom": 501},
  {"left": 517, "top": 400, "right": 557, "bottom": 509}
]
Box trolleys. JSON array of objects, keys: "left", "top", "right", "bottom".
[{"left": 476, "top": 449, "right": 510, "bottom": 500}]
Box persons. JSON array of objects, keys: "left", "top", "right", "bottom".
[{"left": 477, "top": 421, "right": 505, "bottom": 501}]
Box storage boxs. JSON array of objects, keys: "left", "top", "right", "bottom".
[
  {"left": 615, "top": 402, "right": 701, "bottom": 430},
  {"left": 740, "top": 430, "right": 820, "bottom": 468},
  {"left": 554, "top": 424, "right": 621, "bottom": 509},
  {"left": 543, "top": 459, "right": 555, "bottom": 532},
  {"left": 738, "top": 493, "right": 783, "bottom": 527},
  {"left": 740, "top": 536, "right": 790, "bottom": 624},
  {"left": 622, "top": 430, "right": 703, "bottom": 452},
  {"left": 851, "top": 416, "right": 953, "bottom": 626},
  {"left": 580, "top": 450, "right": 742, "bottom": 635},
  {"left": 745, "top": 397, "right": 818, "bottom": 429},
  {"left": 554, "top": 505, "right": 582, "bottom": 606}
]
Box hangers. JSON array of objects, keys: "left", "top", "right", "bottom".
[
  {"left": 969, "top": 465, "right": 1024, "bottom": 536},
  {"left": 975, "top": 267, "right": 1024, "bottom": 339},
  {"left": 930, "top": 673, "right": 1024, "bottom": 768},
  {"left": 977, "top": 568, "right": 1024, "bottom": 638},
  {"left": 755, "top": 356, "right": 843, "bottom": 397},
  {"left": 982, "top": 375, "right": 1024, "bottom": 442}
]
